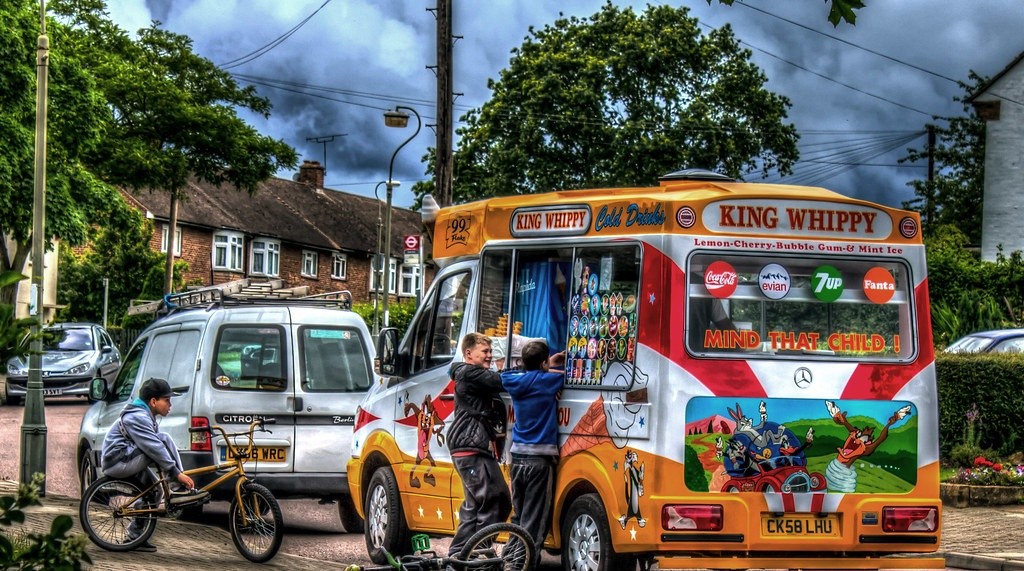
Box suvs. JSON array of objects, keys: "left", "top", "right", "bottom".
[{"left": 77, "top": 288, "right": 380, "bottom": 534}]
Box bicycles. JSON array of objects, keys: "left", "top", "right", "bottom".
[
  {"left": 344, "top": 522, "right": 537, "bottom": 571},
  {"left": 79, "top": 418, "right": 285, "bottom": 564}
]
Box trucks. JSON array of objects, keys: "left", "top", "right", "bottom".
[{"left": 346, "top": 169, "right": 944, "bottom": 571}]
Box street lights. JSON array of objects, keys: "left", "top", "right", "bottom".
[
  {"left": 371, "top": 106, "right": 421, "bottom": 359},
  {"left": 373, "top": 178, "right": 400, "bottom": 360}
]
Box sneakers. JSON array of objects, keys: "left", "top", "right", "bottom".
[
  {"left": 123, "top": 536, "right": 157, "bottom": 552},
  {"left": 169, "top": 485, "right": 208, "bottom": 503}
]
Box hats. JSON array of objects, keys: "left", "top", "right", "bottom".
[{"left": 139, "top": 377, "right": 182, "bottom": 401}]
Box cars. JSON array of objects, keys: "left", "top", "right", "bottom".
[
  {"left": 940, "top": 329, "right": 1024, "bottom": 367},
  {"left": 4, "top": 322, "right": 123, "bottom": 405}
]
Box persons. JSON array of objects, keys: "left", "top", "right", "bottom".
[
  {"left": 440, "top": 332, "right": 566, "bottom": 571},
  {"left": 500, "top": 342, "right": 564, "bottom": 568},
  {"left": 101, "top": 378, "right": 194, "bottom": 553},
  {"left": 400, "top": 272, "right": 470, "bottom": 355}
]
